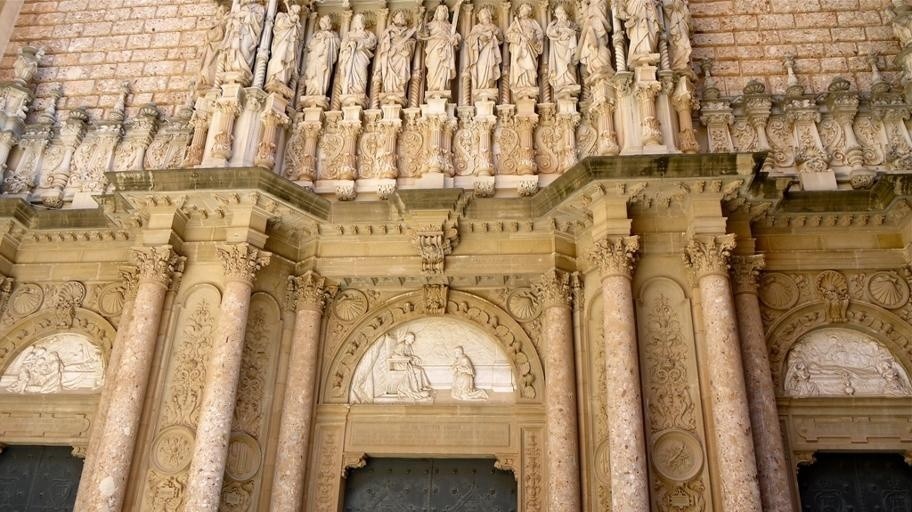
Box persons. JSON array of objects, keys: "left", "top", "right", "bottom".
[
  {"left": 6, "top": 348, "right": 63, "bottom": 394},
  {"left": 194, "top": 0, "right": 692, "bottom": 96},
  {"left": 448, "top": 346, "right": 489, "bottom": 401},
  {"left": 392, "top": 333, "right": 431, "bottom": 400},
  {"left": 784, "top": 336, "right": 906, "bottom": 396}
]
